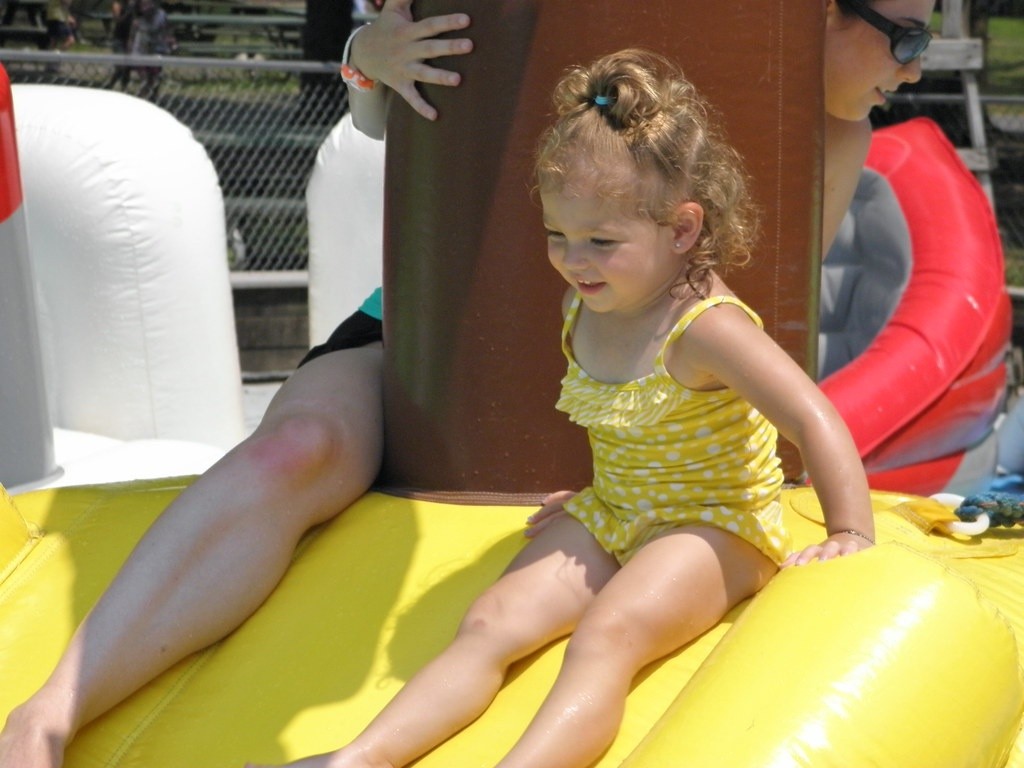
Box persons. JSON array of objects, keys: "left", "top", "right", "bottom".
[
  {"left": 1, "top": 0, "right": 941, "bottom": 767},
  {"left": 243, "top": 47, "right": 878, "bottom": 768},
  {"left": 40, "top": 1, "right": 181, "bottom": 103}
]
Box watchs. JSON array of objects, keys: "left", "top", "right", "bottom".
[{"left": 340, "top": 26, "right": 383, "bottom": 92}]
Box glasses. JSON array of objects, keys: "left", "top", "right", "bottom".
[{"left": 854, "top": 3, "right": 933, "bottom": 65}]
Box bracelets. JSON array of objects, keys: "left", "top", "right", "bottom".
[{"left": 841, "top": 529, "right": 875, "bottom": 545}]
